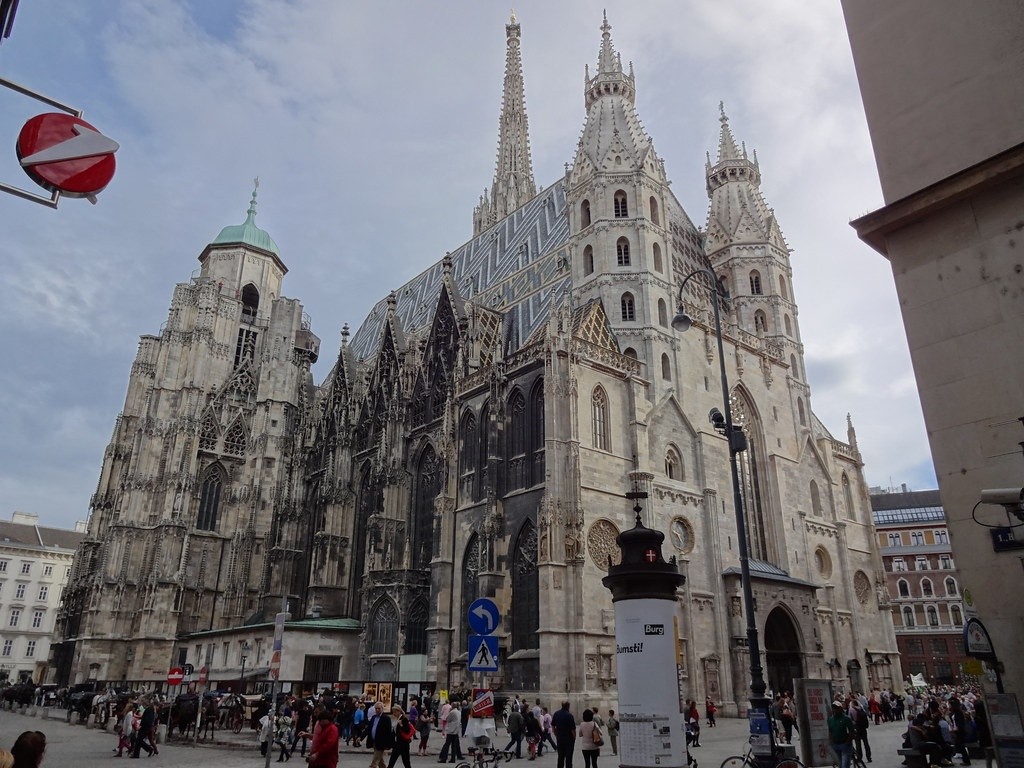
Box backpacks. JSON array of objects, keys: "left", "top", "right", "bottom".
[{"left": 614, "top": 718, "right": 619, "bottom": 731}]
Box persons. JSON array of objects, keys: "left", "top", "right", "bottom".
[{"left": 0, "top": 673, "right": 992, "bottom": 768}]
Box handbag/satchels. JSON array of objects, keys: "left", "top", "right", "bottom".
[
  {"left": 398, "top": 716, "right": 415, "bottom": 741},
  {"left": 416, "top": 715, "right": 427, "bottom": 732},
  {"left": 279, "top": 731, "right": 284, "bottom": 738},
  {"left": 592, "top": 722, "right": 604, "bottom": 745}
]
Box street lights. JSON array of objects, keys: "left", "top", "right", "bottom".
[
  {"left": 239, "top": 643, "right": 250, "bottom": 694},
  {"left": 668, "top": 265, "right": 787, "bottom": 768}
]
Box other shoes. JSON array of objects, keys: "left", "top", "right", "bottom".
[
  {"left": 129, "top": 755, "right": 140, "bottom": 758},
  {"left": 113, "top": 754, "right": 122, "bottom": 757},
  {"left": 153, "top": 750, "right": 159, "bottom": 754},
  {"left": 931, "top": 764, "right": 941, "bottom": 768},
  {"left": 503, "top": 751, "right": 508, "bottom": 758},
  {"left": 457, "top": 756, "right": 466, "bottom": 760},
  {"left": 692, "top": 743, "right": 702, "bottom": 747},
  {"left": 449, "top": 761, "right": 455, "bottom": 763},
  {"left": 515, "top": 756, "right": 523, "bottom": 759},
  {"left": 422, "top": 754, "right": 428, "bottom": 756},
  {"left": 940, "top": 758, "right": 951, "bottom": 764},
  {"left": 437, "top": 760, "right": 446, "bottom": 763},
  {"left": 600, "top": 719, "right": 604, "bottom": 726},
  {"left": 960, "top": 762, "right": 971, "bottom": 766},
  {"left": 416, "top": 753, "right": 422, "bottom": 756},
  {"left": 528, "top": 757, "right": 535, "bottom": 761},
  {"left": 148, "top": 749, "right": 154, "bottom": 757},
  {"left": 537, "top": 754, "right": 543, "bottom": 757}
]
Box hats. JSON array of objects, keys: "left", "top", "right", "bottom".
[
  {"left": 391, "top": 704, "right": 402, "bottom": 710},
  {"left": 832, "top": 700, "right": 841, "bottom": 707}
]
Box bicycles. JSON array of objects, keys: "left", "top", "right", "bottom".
[
  {"left": 453, "top": 744, "right": 514, "bottom": 768},
  {"left": 720, "top": 733, "right": 808, "bottom": 768}
]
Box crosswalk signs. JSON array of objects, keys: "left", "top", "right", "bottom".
[{"left": 468, "top": 635, "right": 500, "bottom": 671}]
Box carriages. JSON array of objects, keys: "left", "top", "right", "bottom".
[{"left": 66, "top": 685, "right": 247, "bottom": 743}]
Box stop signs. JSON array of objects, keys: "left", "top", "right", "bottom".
[
  {"left": 168, "top": 667, "right": 185, "bottom": 685},
  {"left": 199, "top": 668, "right": 208, "bottom": 684}
]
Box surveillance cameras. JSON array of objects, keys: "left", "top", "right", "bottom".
[{"left": 981, "top": 488, "right": 1024, "bottom": 504}]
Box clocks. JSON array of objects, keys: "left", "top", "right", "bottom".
[{"left": 670, "top": 514, "right": 696, "bottom": 554}]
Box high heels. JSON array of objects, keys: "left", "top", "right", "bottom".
[
  {"left": 275, "top": 758, "right": 284, "bottom": 762},
  {"left": 284, "top": 755, "right": 291, "bottom": 762}
]
